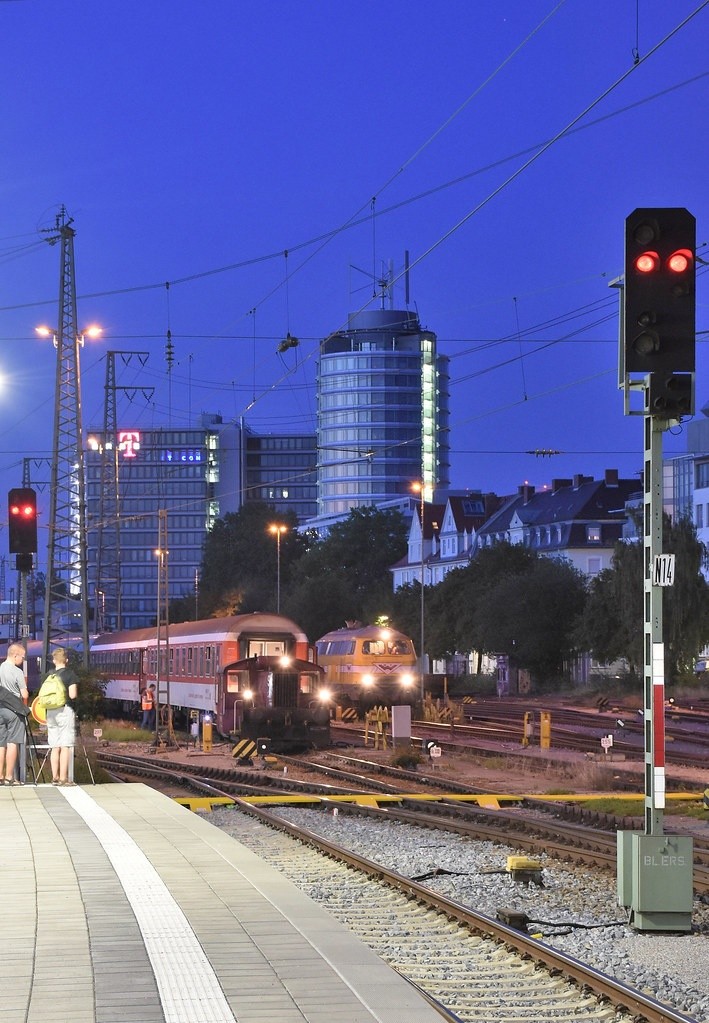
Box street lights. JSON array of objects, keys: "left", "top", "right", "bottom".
[
  {"left": 267, "top": 522, "right": 289, "bottom": 617},
  {"left": 410, "top": 476, "right": 438, "bottom": 703}
]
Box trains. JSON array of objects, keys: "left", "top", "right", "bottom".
[
  {"left": 310, "top": 619, "right": 422, "bottom": 717},
  {"left": 0, "top": 611, "right": 328, "bottom": 750}
]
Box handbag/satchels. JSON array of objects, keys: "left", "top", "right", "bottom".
[{"left": 0, "top": 686, "right": 31, "bottom": 716}]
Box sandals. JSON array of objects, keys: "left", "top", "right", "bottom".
[
  {"left": 0, "top": 779, "right": 24, "bottom": 786},
  {"left": 52, "top": 779, "right": 77, "bottom": 786}
]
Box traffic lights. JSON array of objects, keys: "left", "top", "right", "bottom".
[
  {"left": 628, "top": 207, "right": 699, "bottom": 416},
  {"left": 9, "top": 487, "right": 38, "bottom": 573}
]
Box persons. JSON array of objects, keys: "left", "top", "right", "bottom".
[
  {"left": 0, "top": 643, "right": 29, "bottom": 786},
  {"left": 40, "top": 645, "right": 79, "bottom": 788},
  {"left": 141, "top": 683, "right": 159, "bottom": 730}
]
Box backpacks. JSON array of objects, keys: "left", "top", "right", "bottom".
[{"left": 38, "top": 669, "right": 68, "bottom": 709}]
